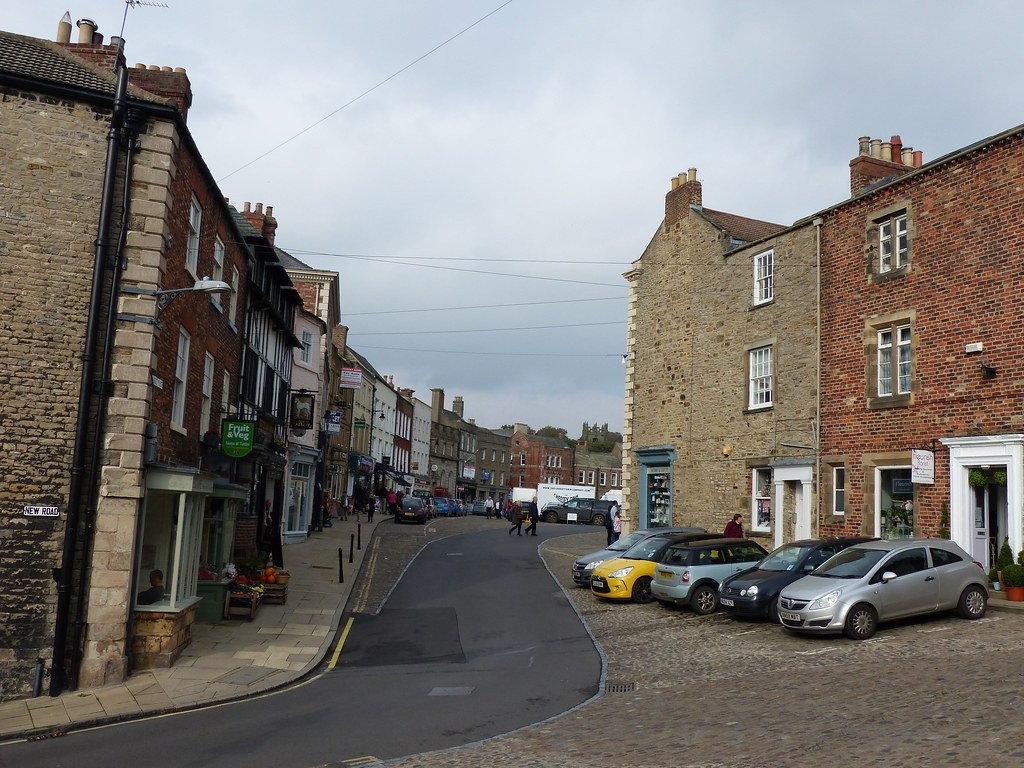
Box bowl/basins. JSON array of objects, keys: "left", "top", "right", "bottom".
[{"left": 274, "top": 574, "right": 290, "bottom": 584}]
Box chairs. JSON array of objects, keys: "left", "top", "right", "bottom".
[
  {"left": 809, "top": 548, "right": 822, "bottom": 561},
  {"left": 702, "top": 549, "right": 746, "bottom": 564}
]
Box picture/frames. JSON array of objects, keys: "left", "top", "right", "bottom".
[{"left": 141, "top": 543, "right": 159, "bottom": 572}]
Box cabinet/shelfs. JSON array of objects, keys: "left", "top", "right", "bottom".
[{"left": 650, "top": 486, "right": 669, "bottom": 526}]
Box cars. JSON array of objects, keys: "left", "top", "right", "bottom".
[
  {"left": 720, "top": 535, "right": 882, "bottom": 623},
  {"left": 571, "top": 526, "right": 707, "bottom": 586},
  {"left": 590, "top": 533, "right": 724, "bottom": 603},
  {"left": 393, "top": 488, "right": 618, "bottom": 525},
  {"left": 777, "top": 537, "right": 989, "bottom": 640},
  {"left": 650, "top": 538, "right": 768, "bottom": 616}
]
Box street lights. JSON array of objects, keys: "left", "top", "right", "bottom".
[{"left": 318, "top": 412, "right": 335, "bottom": 533}]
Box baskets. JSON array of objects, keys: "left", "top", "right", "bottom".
[{"left": 275, "top": 570, "right": 291, "bottom": 584}]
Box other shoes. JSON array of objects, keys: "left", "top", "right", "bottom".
[
  {"left": 525, "top": 529, "right": 528, "bottom": 535},
  {"left": 517, "top": 533, "right": 522, "bottom": 536},
  {"left": 531, "top": 534, "right": 538, "bottom": 536},
  {"left": 509, "top": 530, "right": 511, "bottom": 535}
]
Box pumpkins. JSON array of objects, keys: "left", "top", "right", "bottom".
[{"left": 260, "top": 567, "right": 275, "bottom": 584}]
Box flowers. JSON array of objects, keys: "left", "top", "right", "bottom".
[{"left": 204, "top": 429, "right": 219, "bottom": 438}]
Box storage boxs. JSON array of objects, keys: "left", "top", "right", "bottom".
[{"left": 224, "top": 584, "right": 288, "bottom": 621}]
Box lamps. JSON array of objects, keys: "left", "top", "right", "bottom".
[
  {"left": 372, "top": 410, "right": 386, "bottom": 421},
  {"left": 981, "top": 366, "right": 996, "bottom": 378},
  {"left": 117, "top": 277, "right": 232, "bottom": 330}
]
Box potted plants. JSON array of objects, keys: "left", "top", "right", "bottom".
[
  {"left": 988, "top": 542, "right": 1024, "bottom": 602},
  {"left": 880, "top": 499, "right": 913, "bottom": 540},
  {"left": 275, "top": 437, "right": 289, "bottom": 454}
]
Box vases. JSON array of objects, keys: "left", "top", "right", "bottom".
[{"left": 204, "top": 437, "right": 220, "bottom": 447}]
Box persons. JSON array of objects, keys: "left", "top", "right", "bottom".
[
  {"left": 509, "top": 500, "right": 523, "bottom": 536},
  {"left": 605, "top": 501, "right": 621, "bottom": 546},
  {"left": 136, "top": 570, "right": 165, "bottom": 605},
  {"left": 379, "top": 485, "right": 404, "bottom": 515},
  {"left": 724, "top": 514, "right": 744, "bottom": 538},
  {"left": 506, "top": 499, "right": 513, "bottom": 519},
  {"left": 524, "top": 496, "right": 538, "bottom": 536},
  {"left": 483, "top": 496, "right": 502, "bottom": 519},
  {"left": 367, "top": 495, "right": 376, "bottom": 523},
  {"left": 340, "top": 491, "right": 349, "bottom": 521}
]
